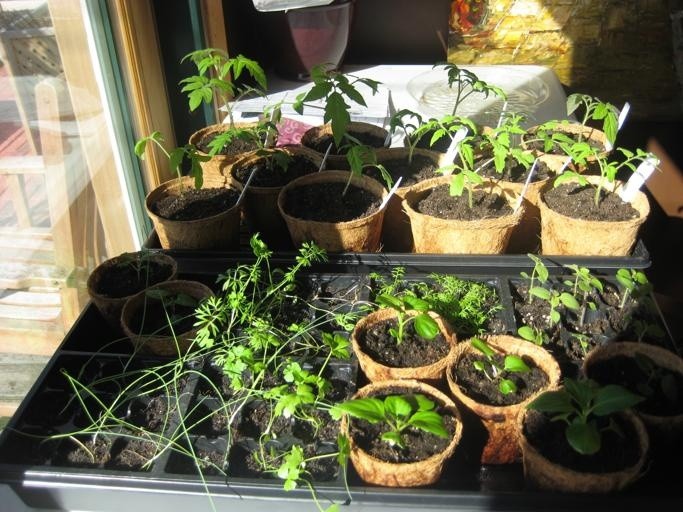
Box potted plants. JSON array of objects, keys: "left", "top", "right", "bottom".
[
  {"left": 537, "top": 132, "right": 652, "bottom": 256},
  {"left": 446, "top": 333, "right": 560, "bottom": 467},
  {"left": 180, "top": 49, "right": 621, "bottom": 226},
  {"left": 516, "top": 374, "right": 651, "bottom": 496},
  {"left": 581, "top": 340, "right": 681, "bottom": 427},
  {"left": 89, "top": 246, "right": 179, "bottom": 316},
  {"left": 277, "top": 131, "right": 387, "bottom": 253},
  {"left": 120, "top": 280, "right": 217, "bottom": 356},
  {"left": 403, "top": 119, "right": 525, "bottom": 253},
  {"left": 133, "top": 126, "right": 248, "bottom": 248},
  {"left": 350, "top": 290, "right": 456, "bottom": 382},
  {"left": 328, "top": 378, "right": 462, "bottom": 489}
]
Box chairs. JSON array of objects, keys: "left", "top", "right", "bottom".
[{"left": 0, "top": 74, "right": 107, "bottom": 419}]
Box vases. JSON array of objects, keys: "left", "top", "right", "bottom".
[{"left": 262, "top": 2, "right": 350, "bottom": 83}]
